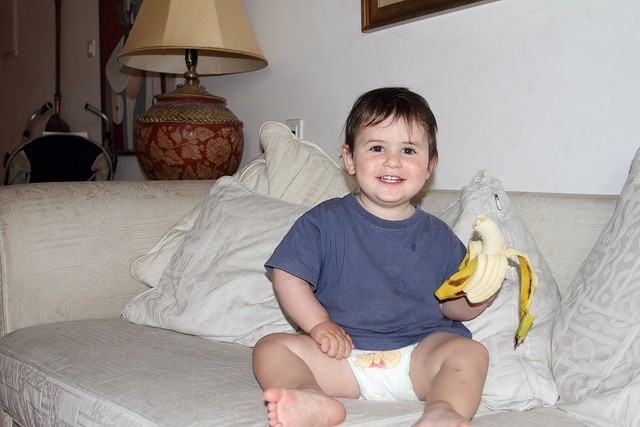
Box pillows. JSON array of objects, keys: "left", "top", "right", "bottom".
[
  {"left": 551, "top": 148, "right": 639, "bottom": 427},
  {"left": 130, "top": 120, "right": 360, "bottom": 288},
  {"left": 120, "top": 176, "right": 315, "bottom": 347},
  {"left": 438, "top": 170, "right": 561, "bottom": 417}
]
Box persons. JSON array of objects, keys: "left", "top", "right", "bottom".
[{"left": 252, "top": 86, "right": 499, "bottom": 426}]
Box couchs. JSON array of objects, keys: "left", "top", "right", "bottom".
[{"left": 0, "top": 179, "right": 621, "bottom": 426}]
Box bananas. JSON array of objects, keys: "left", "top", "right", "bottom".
[{"left": 434, "top": 217, "right": 536, "bottom": 350}]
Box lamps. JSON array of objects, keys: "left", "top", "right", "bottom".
[{"left": 116, "top": 0, "right": 268, "bottom": 180}]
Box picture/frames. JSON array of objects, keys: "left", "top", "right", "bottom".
[{"left": 360, "top": 0, "right": 501, "bottom": 32}]
